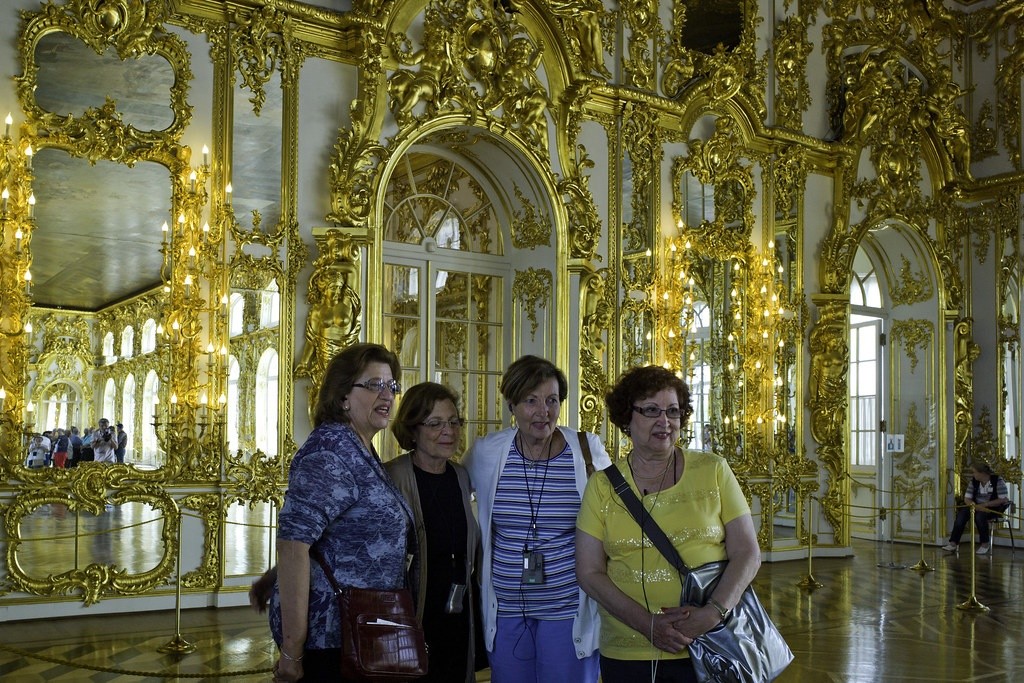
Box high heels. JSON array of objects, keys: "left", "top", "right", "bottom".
[
  {"left": 976, "top": 544, "right": 990, "bottom": 554},
  {"left": 941, "top": 544, "right": 960, "bottom": 552}
]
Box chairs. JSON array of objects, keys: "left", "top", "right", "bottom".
[{"left": 954, "top": 492, "right": 1016, "bottom": 556}]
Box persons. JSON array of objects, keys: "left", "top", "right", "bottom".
[
  {"left": 383, "top": 381, "right": 491, "bottom": 683},
  {"left": 575, "top": 364, "right": 763, "bottom": 683},
  {"left": 942, "top": 459, "right": 1008, "bottom": 554},
  {"left": 270, "top": 343, "right": 422, "bottom": 683},
  {"left": 458, "top": 354, "right": 612, "bottom": 683},
  {"left": 26, "top": 418, "right": 128, "bottom": 468}
]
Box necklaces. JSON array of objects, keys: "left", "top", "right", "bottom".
[
  {"left": 629, "top": 445, "right": 677, "bottom": 479},
  {"left": 520, "top": 431, "right": 551, "bottom": 470}
]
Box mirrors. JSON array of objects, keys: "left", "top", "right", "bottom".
[
  {"left": 672, "top": 114, "right": 757, "bottom": 469},
  {"left": 210, "top": 0, "right": 310, "bottom": 576},
  {"left": 11, "top": 1, "right": 195, "bottom": 606}
]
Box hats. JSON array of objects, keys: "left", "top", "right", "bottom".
[{"left": 116, "top": 424, "right": 124, "bottom": 428}]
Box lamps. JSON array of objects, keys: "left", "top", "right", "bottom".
[
  {"left": 0, "top": 112, "right": 35, "bottom": 483},
  {"left": 152, "top": 144, "right": 232, "bottom": 483},
  {"left": 640, "top": 219, "right": 696, "bottom": 450},
  {"left": 724, "top": 239, "right": 784, "bottom": 453}
]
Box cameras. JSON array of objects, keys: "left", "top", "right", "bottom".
[{"left": 102, "top": 428, "right": 112, "bottom": 442}]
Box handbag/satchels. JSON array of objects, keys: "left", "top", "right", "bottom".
[
  {"left": 679, "top": 559, "right": 795, "bottom": 683},
  {"left": 338, "top": 587, "right": 429, "bottom": 676},
  {"left": 32, "top": 459, "right": 44, "bottom": 466}
]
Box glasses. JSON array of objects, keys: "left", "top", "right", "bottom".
[
  {"left": 634, "top": 406, "right": 686, "bottom": 419},
  {"left": 100, "top": 419, "right": 109, "bottom": 422},
  {"left": 77, "top": 431, "right": 80, "bottom": 433},
  {"left": 351, "top": 377, "right": 401, "bottom": 393},
  {"left": 418, "top": 417, "right": 462, "bottom": 431}
]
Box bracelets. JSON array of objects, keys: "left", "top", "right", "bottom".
[
  {"left": 707, "top": 598, "right": 731, "bottom": 622},
  {"left": 278, "top": 645, "right": 304, "bottom": 662}
]
